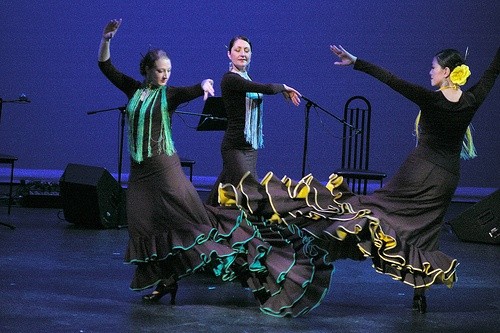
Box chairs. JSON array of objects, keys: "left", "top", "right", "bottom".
[{"left": 330, "top": 95, "right": 387, "bottom": 196}]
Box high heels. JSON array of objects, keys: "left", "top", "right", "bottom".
[
  {"left": 409, "top": 287, "right": 430, "bottom": 317},
  {"left": 141, "top": 278, "right": 181, "bottom": 306}
]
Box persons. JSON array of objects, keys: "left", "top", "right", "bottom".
[
  {"left": 98, "top": 18, "right": 255, "bottom": 306},
  {"left": 206, "top": 36, "right": 302, "bottom": 288},
  {"left": 306, "top": 45, "right": 500, "bottom": 313}
]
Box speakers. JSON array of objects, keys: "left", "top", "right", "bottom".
[
  {"left": 449, "top": 188, "right": 500, "bottom": 245},
  {"left": 59, "top": 163, "right": 128, "bottom": 229}
]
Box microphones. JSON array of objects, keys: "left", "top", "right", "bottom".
[
  {"left": 206, "top": 117, "right": 227, "bottom": 122},
  {"left": 19, "top": 97, "right": 31, "bottom": 103}
]
